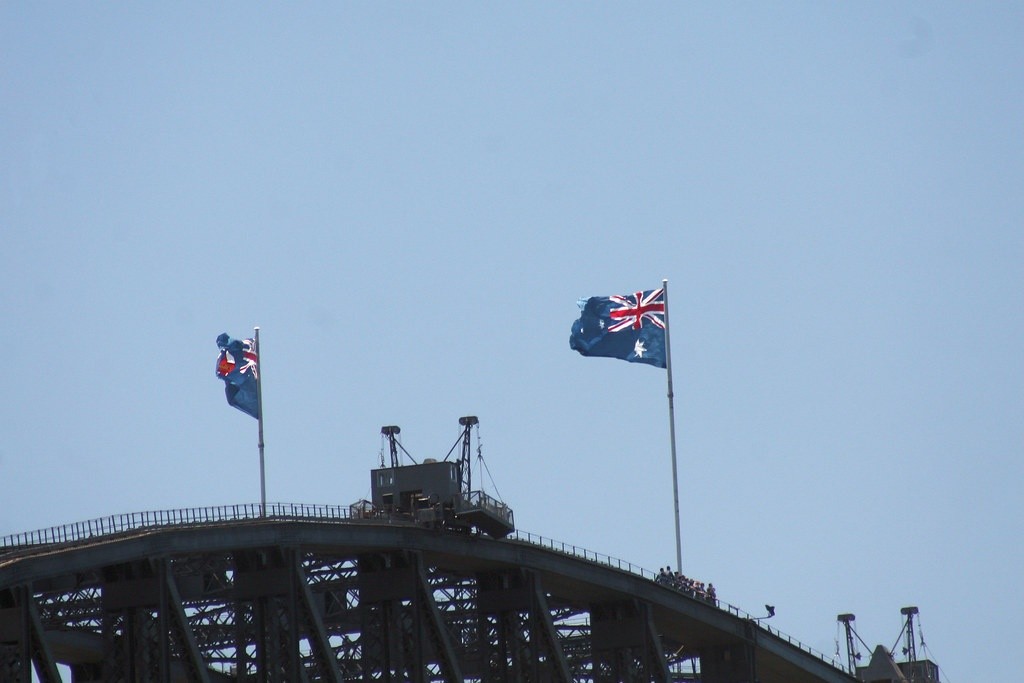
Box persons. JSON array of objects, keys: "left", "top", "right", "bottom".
[{"left": 655, "top": 565, "right": 716, "bottom": 605}]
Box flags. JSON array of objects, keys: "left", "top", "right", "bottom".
[
  {"left": 568, "top": 287, "right": 668, "bottom": 369},
  {"left": 214, "top": 332, "right": 262, "bottom": 419}
]
